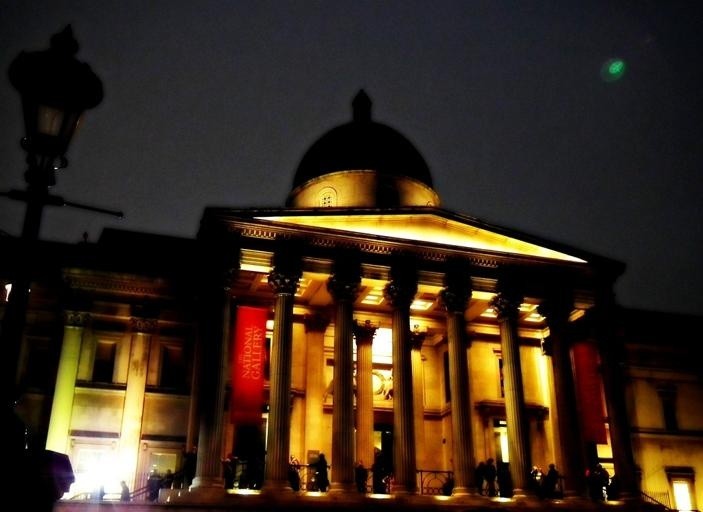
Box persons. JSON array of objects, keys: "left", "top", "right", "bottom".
[
  {"left": 437, "top": 457, "right": 623, "bottom": 500},
  {"left": 89, "top": 451, "right": 196, "bottom": 502},
  {"left": 308, "top": 453, "right": 328, "bottom": 491},
  {"left": 222, "top": 452, "right": 239, "bottom": 489},
  {"left": 355, "top": 463, "right": 369, "bottom": 494}
]
